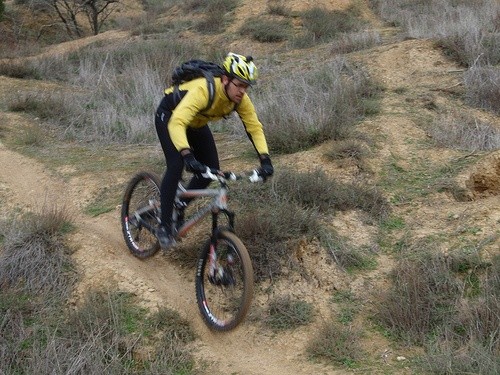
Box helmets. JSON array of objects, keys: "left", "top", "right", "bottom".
[{"left": 221, "top": 51, "right": 258, "bottom": 84}]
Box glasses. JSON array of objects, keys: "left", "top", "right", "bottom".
[{"left": 230, "top": 80, "right": 249, "bottom": 89}]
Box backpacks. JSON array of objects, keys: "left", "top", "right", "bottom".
[{"left": 170, "top": 60, "right": 238, "bottom": 118}]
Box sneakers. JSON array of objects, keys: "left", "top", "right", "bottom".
[
  {"left": 154, "top": 224, "right": 172, "bottom": 249},
  {"left": 175, "top": 224, "right": 188, "bottom": 239}
]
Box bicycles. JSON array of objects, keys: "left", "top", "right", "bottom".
[{"left": 121, "top": 165, "right": 265, "bottom": 332}]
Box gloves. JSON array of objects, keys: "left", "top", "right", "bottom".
[
  {"left": 258, "top": 158, "right": 274, "bottom": 183},
  {"left": 182, "top": 153, "right": 207, "bottom": 173}
]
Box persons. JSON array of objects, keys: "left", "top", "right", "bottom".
[{"left": 154, "top": 53, "right": 274, "bottom": 250}]
